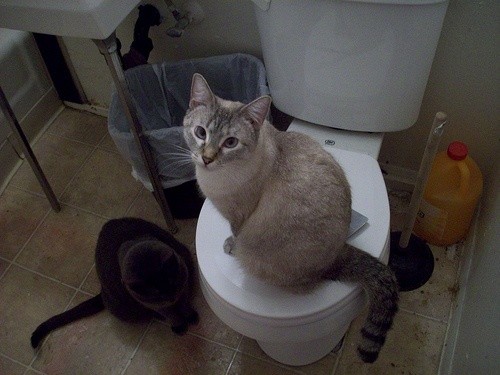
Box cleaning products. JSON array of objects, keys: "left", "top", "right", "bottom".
[{"left": 413, "top": 139, "right": 485, "bottom": 247}]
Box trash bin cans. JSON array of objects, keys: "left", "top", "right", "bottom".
[{"left": 108, "top": 53, "right": 270, "bottom": 225}]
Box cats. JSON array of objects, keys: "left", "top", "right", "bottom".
[
  {"left": 30, "top": 217, "right": 201, "bottom": 350},
  {"left": 183, "top": 73, "right": 401, "bottom": 365}
]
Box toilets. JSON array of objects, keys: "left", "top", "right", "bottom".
[{"left": 194, "top": 1, "right": 453, "bottom": 367}]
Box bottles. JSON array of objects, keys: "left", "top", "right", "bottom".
[{"left": 413, "top": 139, "right": 485, "bottom": 246}]
